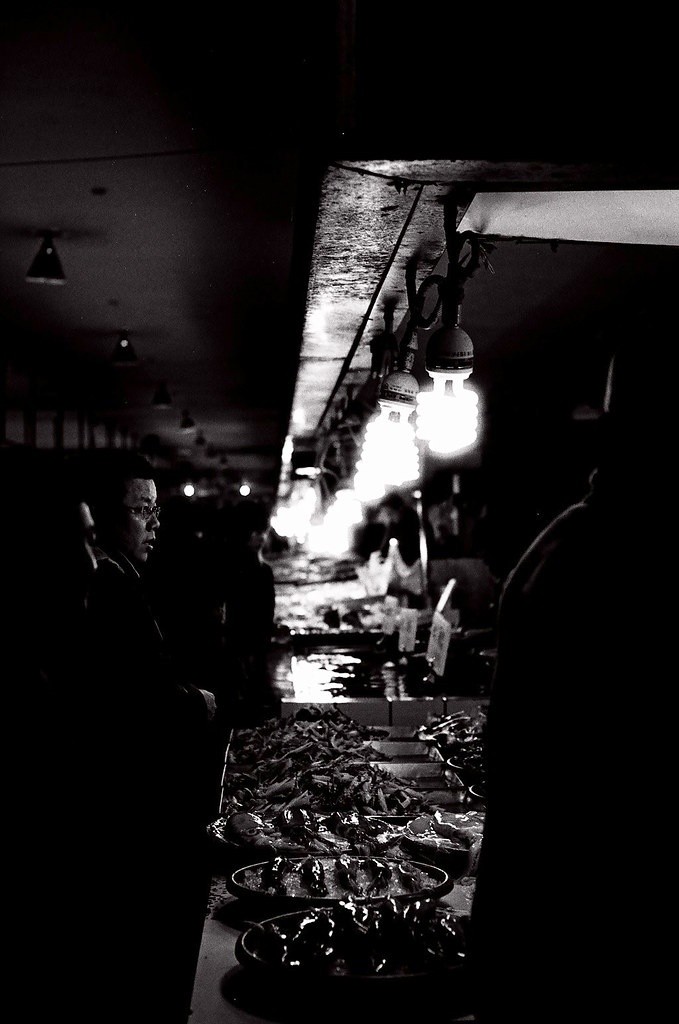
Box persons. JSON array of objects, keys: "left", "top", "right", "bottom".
[
  {"left": 29, "top": 447, "right": 221, "bottom": 1024},
  {"left": 465, "top": 350, "right": 616, "bottom": 1024}
]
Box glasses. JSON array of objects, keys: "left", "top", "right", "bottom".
[{"left": 126, "top": 505, "right": 161, "bottom": 519}]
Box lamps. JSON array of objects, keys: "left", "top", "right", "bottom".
[
  {"left": 105, "top": 328, "right": 139, "bottom": 371},
  {"left": 150, "top": 376, "right": 173, "bottom": 411},
  {"left": 182, "top": 409, "right": 237, "bottom": 467},
  {"left": 26, "top": 229, "right": 70, "bottom": 288}
]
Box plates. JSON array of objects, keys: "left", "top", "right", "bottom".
[
  {"left": 400, "top": 814, "right": 485, "bottom": 859},
  {"left": 205, "top": 814, "right": 397, "bottom": 856},
  {"left": 467, "top": 784, "right": 486, "bottom": 802},
  {"left": 235, "top": 905, "right": 474, "bottom": 992},
  {"left": 225, "top": 858, "right": 454, "bottom": 913},
  {"left": 447, "top": 756, "right": 484, "bottom": 773}
]
